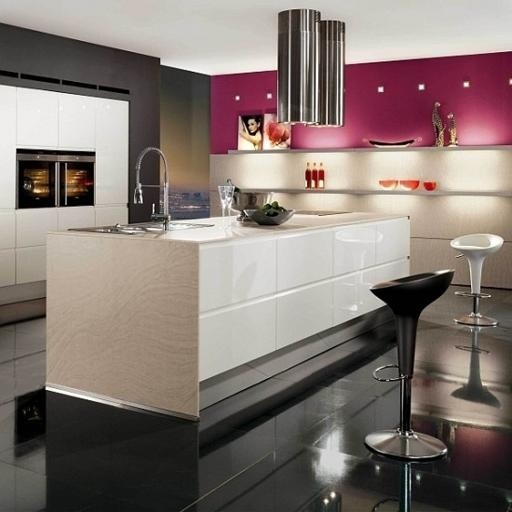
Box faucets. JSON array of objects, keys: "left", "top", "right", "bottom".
[{"left": 134, "top": 147, "right": 170, "bottom": 231}]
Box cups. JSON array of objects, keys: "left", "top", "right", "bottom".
[{"left": 217, "top": 185, "right": 235, "bottom": 222}]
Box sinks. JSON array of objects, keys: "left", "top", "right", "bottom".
[{"left": 73, "top": 222, "right": 215, "bottom": 234}]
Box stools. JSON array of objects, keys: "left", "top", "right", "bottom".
[
  {"left": 363, "top": 270, "right": 449, "bottom": 464},
  {"left": 450, "top": 234, "right": 505, "bottom": 328},
  {"left": 372, "top": 463, "right": 450, "bottom": 512},
  {"left": 450, "top": 328, "right": 505, "bottom": 410}
]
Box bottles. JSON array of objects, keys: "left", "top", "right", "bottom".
[
  {"left": 305, "top": 162, "right": 312, "bottom": 189},
  {"left": 311, "top": 163, "right": 318, "bottom": 188},
  {"left": 319, "top": 163, "right": 325, "bottom": 189}
]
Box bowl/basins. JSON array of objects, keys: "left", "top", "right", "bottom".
[
  {"left": 379, "top": 180, "right": 398, "bottom": 191},
  {"left": 423, "top": 182, "right": 437, "bottom": 191},
  {"left": 401, "top": 180, "right": 420, "bottom": 191}
]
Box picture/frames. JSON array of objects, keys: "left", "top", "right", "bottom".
[
  {"left": 263, "top": 113, "right": 293, "bottom": 151},
  {"left": 237, "top": 115, "right": 262, "bottom": 151}
]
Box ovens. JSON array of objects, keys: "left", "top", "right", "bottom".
[
  {"left": 14, "top": 386, "right": 45, "bottom": 461},
  {"left": 15, "top": 148, "right": 96, "bottom": 210}
]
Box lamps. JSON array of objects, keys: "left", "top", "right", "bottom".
[
  {"left": 277, "top": 8, "right": 322, "bottom": 127},
  {"left": 318, "top": 17, "right": 346, "bottom": 127}
]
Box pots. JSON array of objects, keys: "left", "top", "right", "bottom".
[{"left": 230, "top": 191, "right": 276, "bottom": 221}]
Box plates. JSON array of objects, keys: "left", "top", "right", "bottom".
[{"left": 251, "top": 210, "right": 296, "bottom": 225}]
